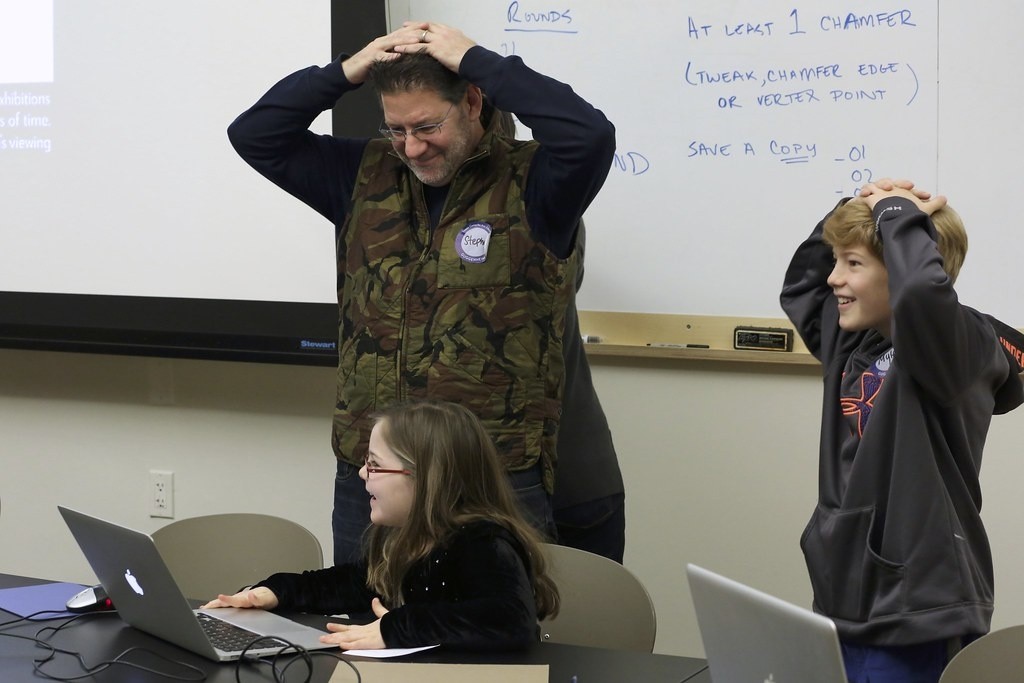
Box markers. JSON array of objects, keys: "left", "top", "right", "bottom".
[
  {"left": 581, "top": 336, "right": 601, "bottom": 343},
  {"left": 647, "top": 343, "right": 710, "bottom": 349}
]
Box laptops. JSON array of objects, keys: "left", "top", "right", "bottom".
[
  {"left": 57, "top": 505, "right": 339, "bottom": 660},
  {"left": 686, "top": 562, "right": 848, "bottom": 683}
]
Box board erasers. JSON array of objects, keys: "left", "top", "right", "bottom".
[{"left": 732, "top": 326, "right": 794, "bottom": 353}]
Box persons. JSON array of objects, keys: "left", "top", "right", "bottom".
[
  {"left": 226, "top": 19, "right": 617, "bottom": 570},
  {"left": 779, "top": 178, "right": 1024, "bottom": 683},
  {"left": 501, "top": 112, "right": 626, "bottom": 566},
  {"left": 198, "top": 397, "right": 562, "bottom": 651}
]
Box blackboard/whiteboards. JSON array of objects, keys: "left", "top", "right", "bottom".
[{"left": 384, "top": 0, "right": 1023, "bottom": 366}]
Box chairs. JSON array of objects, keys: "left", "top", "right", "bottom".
[
  {"left": 151, "top": 513, "right": 324, "bottom": 602},
  {"left": 537, "top": 542, "right": 657, "bottom": 655},
  {"left": 940, "top": 625, "right": 1024, "bottom": 683}
]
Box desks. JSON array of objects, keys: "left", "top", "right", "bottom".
[{"left": 0, "top": 573, "right": 709, "bottom": 683}]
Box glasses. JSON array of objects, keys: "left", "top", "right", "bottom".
[
  {"left": 378, "top": 102, "right": 454, "bottom": 142},
  {"left": 361, "top": 454, "right": 414, "bottom": 481}
]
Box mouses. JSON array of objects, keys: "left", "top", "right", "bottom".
[{"left": 66, "top": 584, "right": 111, "bottom": 613}]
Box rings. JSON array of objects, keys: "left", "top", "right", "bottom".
[{"left": 422, "top": 29, "right": 429, "bottom": 41}]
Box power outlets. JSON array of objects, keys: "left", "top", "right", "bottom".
[{"left": 148, "top": 470, "right": 174, "bottom": 518}]
